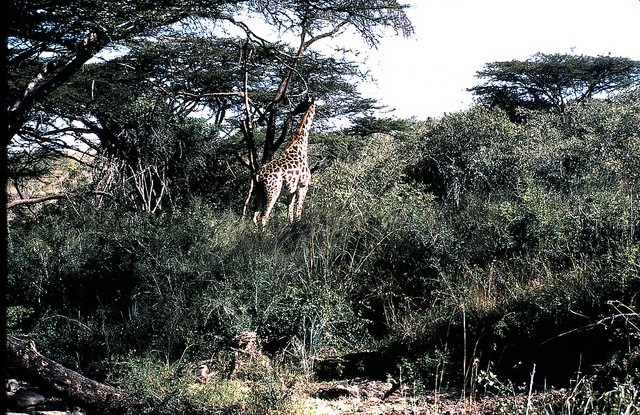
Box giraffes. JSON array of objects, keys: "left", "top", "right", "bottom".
[{"left": 235, "top": 97, "right": 317, "bottom": 237}]
